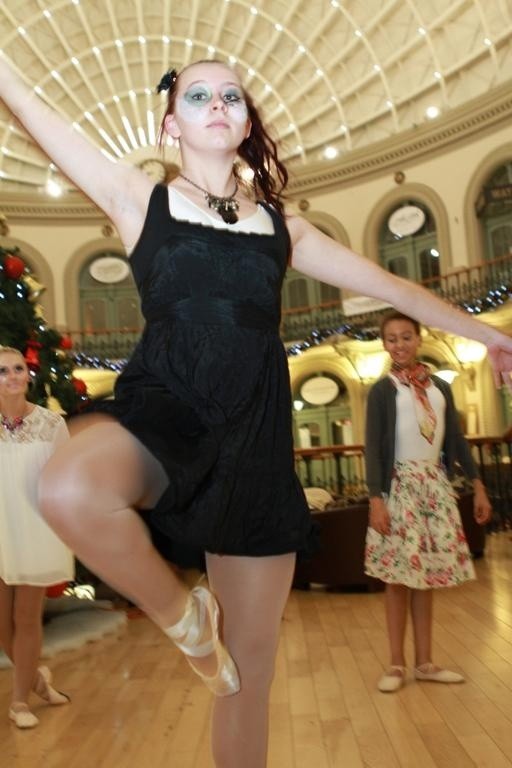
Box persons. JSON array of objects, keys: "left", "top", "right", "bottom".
[
  {"left": 359, "top": 312, "right": 492, "bottom": 694},
  {"left": 0, "top": 346, "right": 71, "bottom": 728},
  {"left": 0, "top": 52, "right": 512, "bottom": 768}
]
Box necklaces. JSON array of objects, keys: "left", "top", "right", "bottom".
[
  {"left": 0, "top": 400, "right": 30, "bottom": 431},
  {"left": 179, "top": 173, "right": 243, "bottom": 225}
]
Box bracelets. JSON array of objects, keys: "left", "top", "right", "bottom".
[{"left": 472, "top": 483, "right": 486, "bottom": 490}]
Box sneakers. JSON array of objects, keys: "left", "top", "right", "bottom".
[
  {"left": 377, "top": 665, "right": 406, "bottom": 692},
  {"left": 7, "top": 701, "right": 39, "bottom": 728},
  {"left": 159, "top": 586, "right": 240, "bottom": 697},
  {"left": 412, "top": 663, "right": 464, "bottom": 684},
  {"left": 35, "top": 664, "right": 71, "bottom": 704}
]
